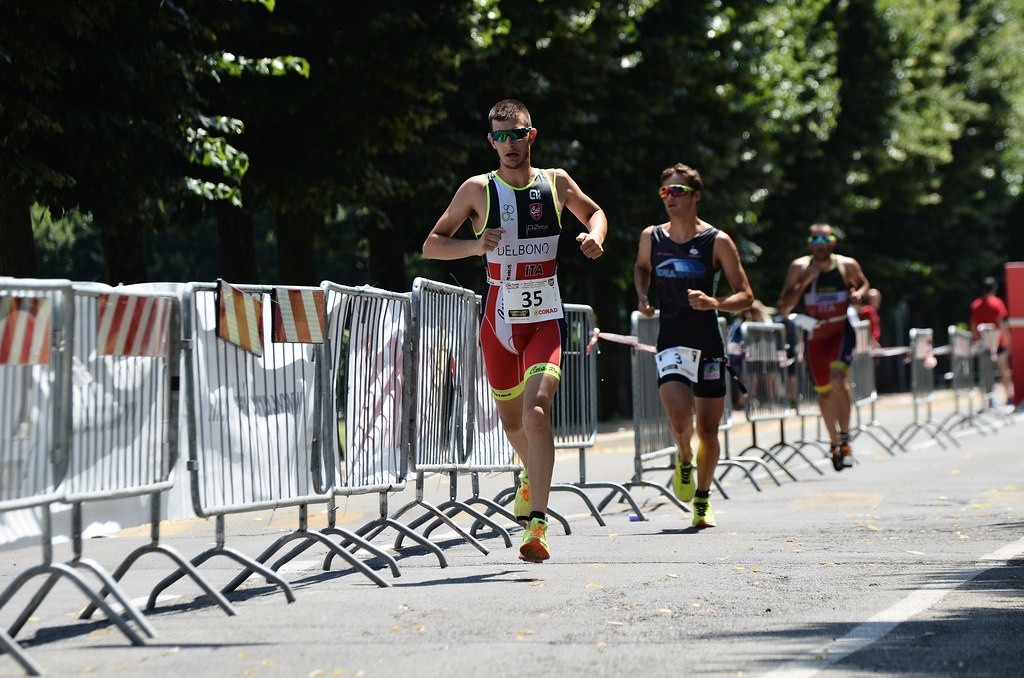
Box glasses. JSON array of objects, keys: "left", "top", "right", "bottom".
[
  {"left": 491, "top": 127, "right": 532, "bottom": 142},
  {"left": 807, "top": 236, "right": 834, "bottom": 245},
  {"left": 658, "top": 184, "right": 696, "bottom": 199}
]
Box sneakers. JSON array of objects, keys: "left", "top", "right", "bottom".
[
  {"left": 519, "top": 518, "right": 550, "bottom": 563},
  {"left": 691, "top": 497, "right": 717, "bottom": 527},
  {"left": 673, "top": 445, "right": 696, "bottom": 503},
  {"left": 514, "top": 468, "right": 530, "bottom": 522},
  {"left": 840, "top": 444, "right": 853, "bottom": 467},
  {"left": 830, "top": 444, "right": 845, "bottom": 471}
]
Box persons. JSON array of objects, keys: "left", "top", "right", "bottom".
[
  {"left": 726, "top": 310, "right": 756, "bottom": 410},
  {"left": 635, "top": 163, "right": 754, "bottom": 531},
  {"left": 970, "top": 277, "right": 1014, "bottom": 405},
  {"left": 423, "top": 100, "right": 608, "bottom": 564},
  {"left": 846, "top": 303, "right": 871, "bottom": 400},
  {"left": 746, "top": 299, "right": 778, "bottom": 410},
  {"left": 772, "top": 303, "right": 804, "bottom": 409},
  {"left": 777, "top": 221, "right": 869, "bottom": 471},
  {"left": 857, "top": 288, "right": 881, "bottom": 348}
]
